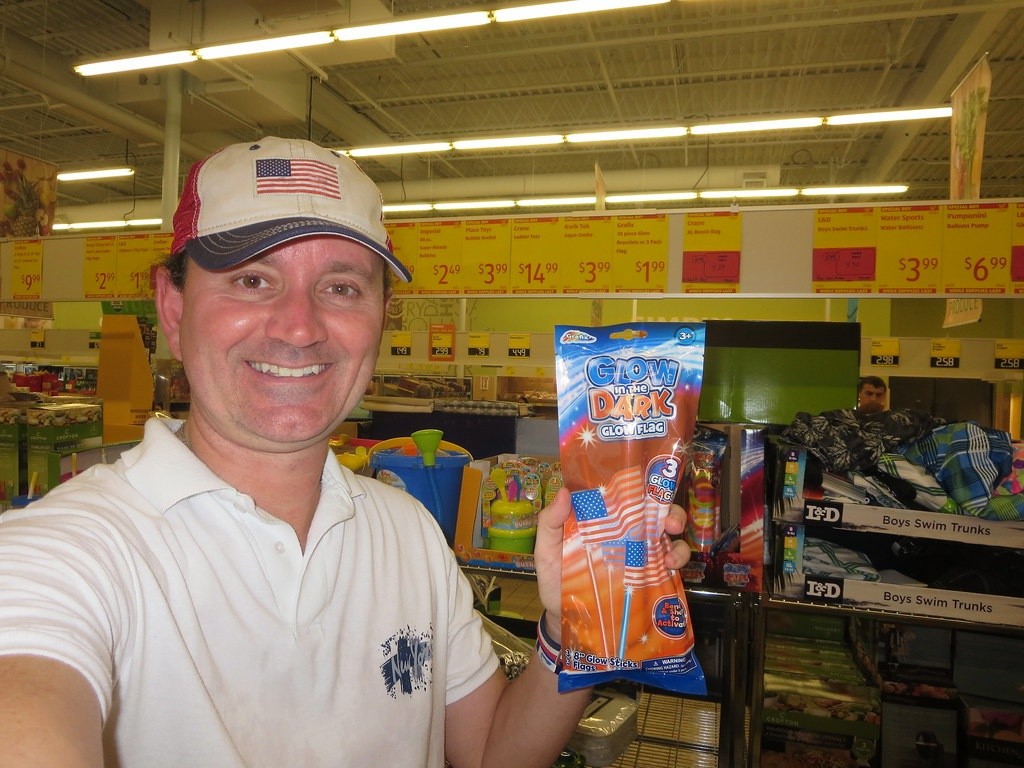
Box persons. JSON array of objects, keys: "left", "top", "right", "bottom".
[
  {"left": 857, "top": 375, "right": 885, "bottom": 413},
  {"left": 1, "top": 136, "right": 696, "bottom": 766}
]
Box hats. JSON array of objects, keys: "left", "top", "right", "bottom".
[{"left": 161, "top": 132, "right": 415, "bottom": 281}]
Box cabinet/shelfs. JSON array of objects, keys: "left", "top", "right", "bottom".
[{"left": 1, "top": 371, "right": 1024, "bottom": 768}]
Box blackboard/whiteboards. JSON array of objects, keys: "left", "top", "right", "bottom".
[
  {"left": 860, "top": 336, "right": 1022, "bottom": 382},
  {"left": 373, "top": 329, "right": 591, "bottom": 369},
  {"left": 1, "top": 196, "right": 1024, "bottom": 299},
  {"left": 0, "top": 326, "right": 118, "bottom": 366}
]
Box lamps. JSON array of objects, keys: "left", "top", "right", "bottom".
[
  {"left": 58, "top": 166, "right": 136, "bottom": 182},
  {"left": 71, "top": 0, "right": 671, "bottom": 79},
  {"left": 335, "top": 103, "right": 955, "bottom": 160},
  {"left": 381, "top": 182, "right": 910, "bottom": 213},
  {"left": 52, "top": 218, "right": 162, "bottom": 229}
]
